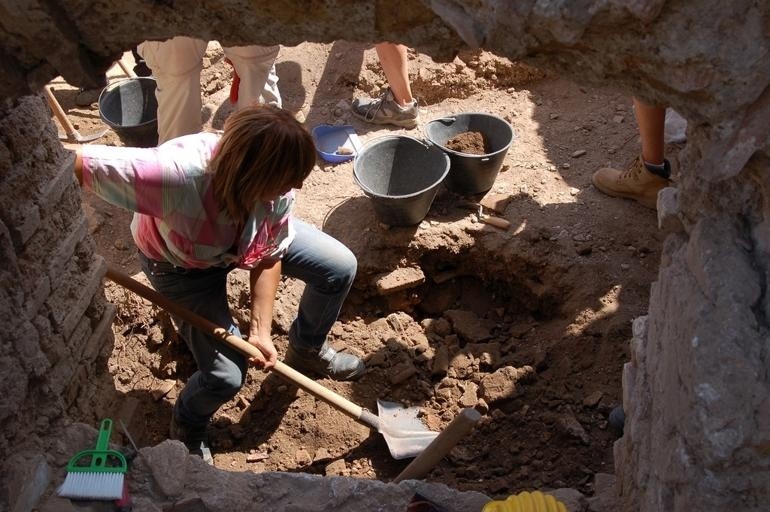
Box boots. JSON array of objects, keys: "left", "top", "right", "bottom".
[{"left": 592, "top": 154, "right": 671, "bottom": 210}]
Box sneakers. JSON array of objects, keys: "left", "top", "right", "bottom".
[{"left": 351, "top": 87, "right": 418, "bottom": 130}]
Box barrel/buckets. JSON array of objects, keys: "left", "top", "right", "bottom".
[
  {"left": 97, "top": 77, "right": 159, "bottom": 148},
  {"left": 351, "top": 133, "right": 451, "bottom": 228},
  {"left": 424, "top": 111, "right": 516, "bottom": 195}
]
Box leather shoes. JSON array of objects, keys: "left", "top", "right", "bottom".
[
  {"left": 286, "top": 344, "right": 366, "bottom": 381},
  {"left": 169, "top": 419, "right": 214, "bottom": 466},
  {"left": 76, "top": 86, "right": 104, "bottom": 106}
]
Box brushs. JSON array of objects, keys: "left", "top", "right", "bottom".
[{"left": 57, "top": 418, "right": 130, "bottom": 512}]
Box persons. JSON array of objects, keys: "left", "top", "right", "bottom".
[
  {"left": 352, "top": 42, "right": 420, "bottom": 130},
  {"left": 69, "top": 106, "right": 358, "bottom": 466},
  {"left": 76, "top": 73, "right": 109, "bottom": 107},
  {"left": 137, "top": 40, "right": 282, "bottom": 140},
  {"left": 590, "top": 97, "right": 672, "bottom": 211}
]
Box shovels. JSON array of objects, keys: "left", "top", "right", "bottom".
[
  {"left": 104, "top": 269, "right": 442, "bottom": 459},
  {"left": 456, "top": 202, "right": 511, "bottom": 231},
  {"left": 42, "top": 85, "right": 108, "bottom": 143}
]
